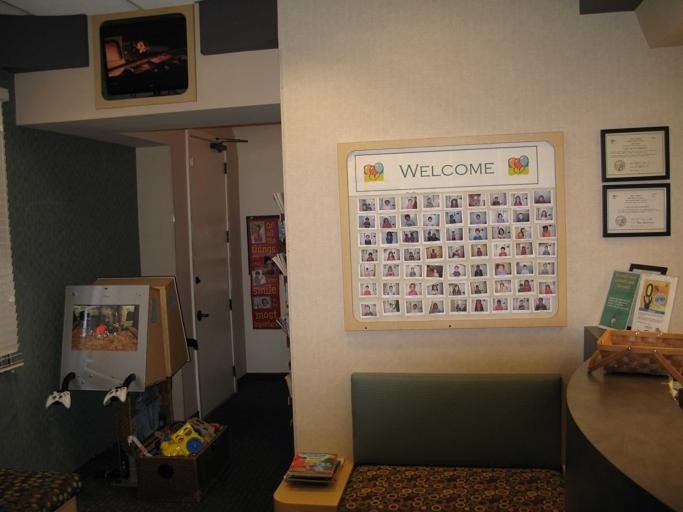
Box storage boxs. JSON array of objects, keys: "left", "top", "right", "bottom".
[{"left": 117, "top": 376, "right": 233, "bottom": 504}]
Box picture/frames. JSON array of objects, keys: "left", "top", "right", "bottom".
[
  {"left": 85, "top": 4, "right": 198, "bottom": 114},
  {"left": 601, "top": 185, "right": 670, "bottom": 238},
  {"left": 333, "top": 129, "right": 572, "bottom": 332},
  {"left": 600, "top": 123, "right": 671, "bottom": 183},
  {"left": 625, "top": 261, "right": 670, "bottom": 329}
]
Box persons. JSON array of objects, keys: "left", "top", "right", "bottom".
[
  {"left": 256, "top": 298, "right": 271, "bottom": 309},
  {"left": 253, "top": 225, "right": 264, "bottom": 242},
  {"left": 253, "top": 271, "right": 263, "bottom": 284},
  {"left": 262, "top": 256, "right": 278, "bottom": 276},
  {"left": 356, "top": 194, "right": 552, "bottom": 315}
]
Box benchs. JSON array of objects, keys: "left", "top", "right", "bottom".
[
  {"left": 336, "top": 363, "right": 568, "bottom": 512},
  {"left": 0, "top": 465, "right": 84, "bottom": 511}
]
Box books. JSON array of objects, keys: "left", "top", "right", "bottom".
[
  {"left": 597, "top": 270, "right": 642, "bottom": 331},
  {"left": 271, "top": 253, "right": 286, "bottom": 274},
  {"left": 284, "top": 459, "right": 341, "bottom": 485},
  {"left": 336, "top": 456, "right": 345, "bottom": 464},
  {"left": 275, "top": 315, "right": 286, "bottom": 333},
  {"left": 271, "top": 190, "right": 282, "bottom": 212},
  {"left": 284, "top": 374, "right": 291, "bottom": 395},
  {"left": 287, "top": 451, "right": 338, "bottom": 478}
]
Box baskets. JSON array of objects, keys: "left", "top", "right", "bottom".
[{"left": 585, "top": 327, "right": 683, "bottom": 379}]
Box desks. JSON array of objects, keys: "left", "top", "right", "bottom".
[
  {"left": 580, "top": 326, "right": 634, "bottom": 364},
  {"left": 272, "top": 456, "right": 355, "bottom": 510}
]
